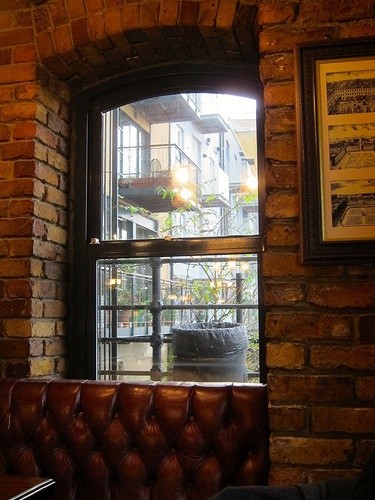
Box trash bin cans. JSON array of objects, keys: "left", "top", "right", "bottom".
[{"left": 171, "top": 321, "right": 250, "bottom": 384}]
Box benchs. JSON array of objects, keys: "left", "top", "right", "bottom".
[{"left": 0, "top": 376, "right": 268, "bottom": 500}]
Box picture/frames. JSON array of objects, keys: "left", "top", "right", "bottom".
[{"left": 294, "top": 36, "right": 375, "bottom": 265}]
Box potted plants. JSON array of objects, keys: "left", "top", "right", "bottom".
[
  {"left": 119, "top": 299, "right": 130, "bottom": 328},
  {"left": 172, "top": 262, "right": 250, "bottom": 383}
]
